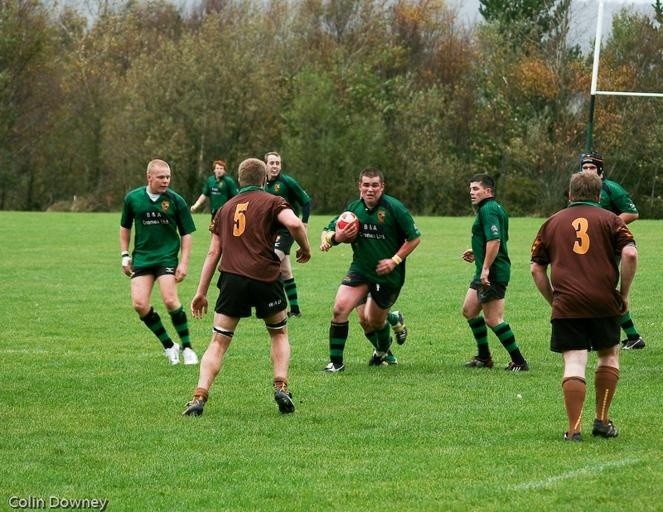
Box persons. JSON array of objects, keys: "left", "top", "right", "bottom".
[
  {"left": 114, "top": 158, "right": 198, "bottom": 367},
  {"left": 179, "top": 157, "right": 312, "bottom": 415},
  {"left": 456, "top": 173, "right": 529, "bottom": 372},
  {"left": 530, "top": 167, "right": 637, "bottom": 442},
  {"left": 322, "top": 166, "right": 422, "bottom": 376},
  {"left": 259, "top": 150, "right": 311, "bottom": 318},
  {"left": 568, "top": 150, "right": 647, "bottom": 352},
  {"left": 318, "top": 190, "right": 408, "bottom": 366},
  {"left": 188, "top": 159, "right": 242, "bottom": 218}
]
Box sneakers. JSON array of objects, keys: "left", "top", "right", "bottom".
[
  {"left": 164, "top": 341, "right": 199, "bottom": 365},
  {"left": 286, "top": 310, "right": 301, "bottom": 317},
  {"left": 325, "top": 361, "right": 345, "bottom": 373},
  {"left": 392, "top": 311, "right": 406, "bottom": 345},
  {"left": 273, "top": 389, "right": 295, "bottom": 414},
  {"left": 563, "top": 431, "right": 582, "bottom": 441},
  {"left": 591, "top": 419, "right": 617, "bottom": 437},
  {"left": 620, "top": 337, "right": 646, "bottom": 350},
  {"left": 504, "top": 360, "right": 529, "bottom": 372},
  {"left": 465, "top": 354, "right": 493, "bottom": 367},
  {"left": 182, "top": 399, "right": 204, "bottom": 416},
  {"left": 369, "top": 349, "right": 396, "bottom": 366}
]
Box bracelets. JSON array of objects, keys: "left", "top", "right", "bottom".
[{"left": 392, "top": 254, "right": 403, "bottom": 267}]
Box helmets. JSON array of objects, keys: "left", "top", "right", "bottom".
[{"left": 579, "top": 152, "right": 604, "bottom": 176}]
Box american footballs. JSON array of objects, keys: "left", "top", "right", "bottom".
[{"left": 338, "top": 210, "right": 359, "bottom": 232}]
